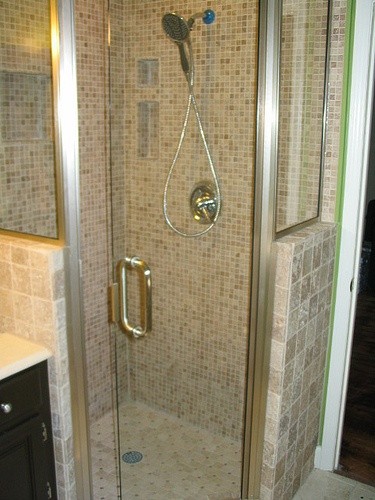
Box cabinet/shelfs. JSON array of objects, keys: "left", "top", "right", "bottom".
[{"left": 1, "top": 360, "right": 61, "bottom": 500}]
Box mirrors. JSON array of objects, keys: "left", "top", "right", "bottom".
[{"left": 1, "top": 0, "right": 65, "bottom": 246}]
